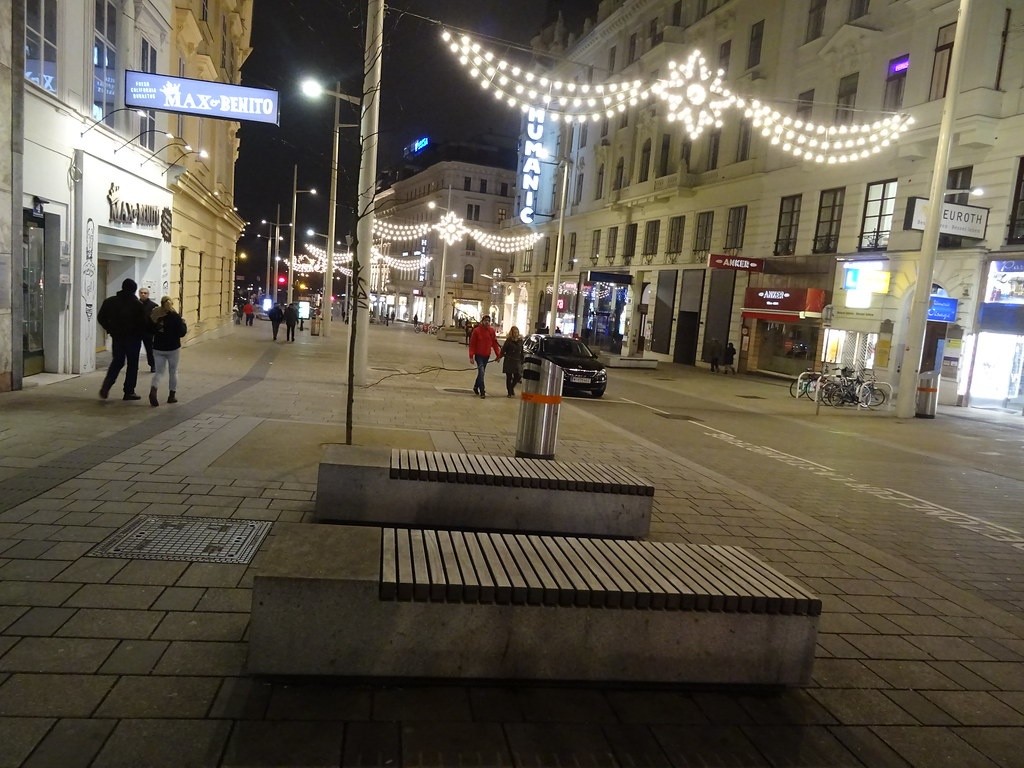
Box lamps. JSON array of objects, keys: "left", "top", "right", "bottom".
[
  {"left": 113, "top": 130, "right": 173, "bottom": 155},
  {"left": 161, "top": 150, "right": 207, "bottom": 173},
  {"left": 140, "top": 142, "right": 193, "bottom": 167},
  {"left": 80, "top": 108, "right": 147, "bottom": 138}
]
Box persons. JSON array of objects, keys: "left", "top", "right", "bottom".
[
  {"left": 284, "top": 303, "right": 299, "bottom": 341},
  {"left": 555, "top": 327, "right": 561, "bottom": 334},
  {"left": 391, "top": 312, "right": 395, "bottom": 323},
  {"left": 454, "top": 313, "right": 469, "bottom": 329},
  {"left": 233, "top": 301, "right": 254, "bottom": 326},
  {"left": 149, "top": 295, "right": 187, "bottom": 407},
  {"left": 497, "top": 326, "right": 524, "bottom": 398},
  {"left": 97, "top": 279, "right": 147, "bottom": 400},
  {"left": 470, "top": 315, "right": 500, "bottom": 399},
  {"left": 466, "top": 322, "right": 474, "bottom": 347},
  {"left": 724, "top": 343, "right": 736, "bottom": 376},
  {"left": 138, "top": 288, "right": 156, "bottom": 373},
  {"left": 414, "top": 315, "right": 418, "bottom": 326},
  {"left": 709, "top": 338, "right": 722, "bottom": 374},
  {"left": 537, "top": 323, "right": 547, "bottom": 334},
  {"left": 269, "top": 303, "right": 284, "bottom": 340},
  {"left": 342, "top": 311, "right": 345, "bottom": 321}
]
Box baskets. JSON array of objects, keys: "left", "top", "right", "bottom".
[{"left": 841, "top": 367, "right": 852, "bottom": 377}]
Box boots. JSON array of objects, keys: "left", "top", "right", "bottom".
[
  {"left": 149, "top": 386, "right": 160, "bottom": 406},
  {"left": 167, "top": 390, "right": 178, "bottom": 403}
]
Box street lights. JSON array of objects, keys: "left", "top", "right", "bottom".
[
  {"left": 536, "top": 123, "right": 572, "bottom": 334},
  {"left": 257, "top": 164, "right": 317, "bottom": 308},
  {"left": 337, "top": 229, "right": 350, "bottom": 322},
  {"left": 427, "top": 184, "right": 451, "bottom": 327}
]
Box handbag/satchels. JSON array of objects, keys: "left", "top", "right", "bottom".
[{"left": 517, "top": 365, "right": 523, "bottom": 384}]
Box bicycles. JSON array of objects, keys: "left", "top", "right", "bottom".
[
  {"left": 789, "top": 360, "right": 889, "bottom": 411},
  {"left": 414, "top": 321, "right": 440, "bottom": 335}
]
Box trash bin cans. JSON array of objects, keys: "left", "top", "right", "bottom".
[
  {"left": 515, "top": 354, "right": 564, "bottom": 460},
  {"left": 311, "top": 314, "right": 320, "bottom": 335},
  {"left": 915, "top": 370, "right": 941, "bottom": 418}
]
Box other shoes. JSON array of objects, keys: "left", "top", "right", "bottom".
[
  {"left": 473, "top": 386, "right": 479, "bottom": 395},
  {"left": 150, "top": 364, "right": 156, "bottom": 373},
  {"left": 291, "top": 338, "right": 295, "bottom": 342},
  {"left": 287, "top": 338, "right": 289, "bottom": 341},
  {"left": 246, "top": 320, "right": 248, "bottom": 326},
  {"left": 99, "top": 388, "right": 108, "bottom": 399},
  {"left": 506, "top": 388, "right": 515, "bottom": 397},
  {"left": 481, "top": 391, "right": 485, "bottom": 398},
  {"left": 122, "top": 393, "right": 141, "bottom": 400}
]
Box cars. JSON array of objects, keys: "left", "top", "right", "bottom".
[{"left": 522, "top": 334, "right": 607, "bottom": 397}]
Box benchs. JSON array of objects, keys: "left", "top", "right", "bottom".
[
  {"left": 245, "top": 523, "right": 824, "bottom": 687},
  {"left": 315, "top": 443, "right": 653, "bottom": 540}
]
